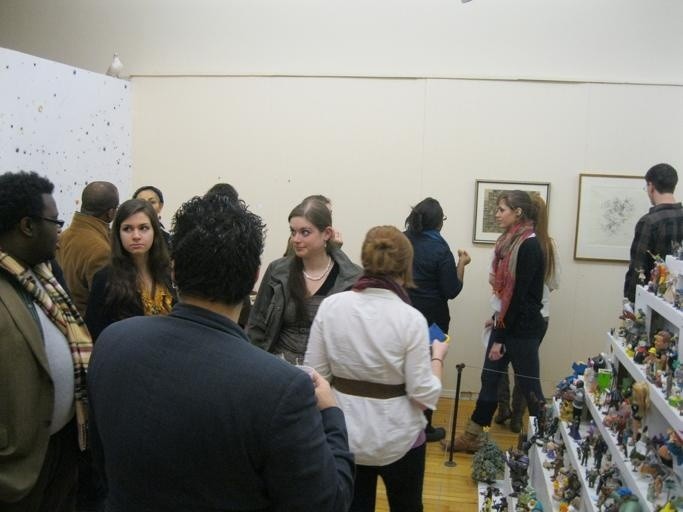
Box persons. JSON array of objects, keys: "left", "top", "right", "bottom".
[
  {"left": 244, "top": 198, "right": 367, "bottom": 370},
  {"left": 84, "top": 199, "right": 178, "bottom": 346},
  {"left": 131, "top": 186, "right": 176, "bottom": 262},
  {"left": 302, "top": 226, "right": 450, "bottom": 511},
  {"left": 85, "top": 194, "right": 356, "bottom": 512},
  {"left": 474, "top": 242, "right": 683, "bottom": 512},
  {"left": 399, "top": 198, "right": 471, "bottom": 442},
  {"left": 207, "top": 182, "right": 254, "bottom": 330},
  {"left": 444, "top": 190, "right": 546, "bottom": 453},
  {"left": 283, "top": 196, "right": 343, "bottom": 259},
  {"left": 52, "top": 181, "right": 119, "bottom": 321},
  {"left": 1, "top": 170, "right": 104, "bottom": 512},
  {"left": 624, "top": 164, "right": 683, "bottom": 315},
  {"left": 483, "top": 191, "right": 560, "bottom": 434},
  {"left": 473, "top": 241, "right": 683, "bottom": 512}
]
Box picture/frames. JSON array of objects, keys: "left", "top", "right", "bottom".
[
  {"left": 472, "top": 179, "right": 550, "bottom": 245},
  {"left": 573, "top": 173, "right": 654, "bottom": 262}
]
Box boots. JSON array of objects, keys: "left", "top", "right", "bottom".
[
  {"left": 495, "top": 372, "right": 525, "bottom": 432},
  {"left": 441, "top": 420, "right": 481, "bottom": 454}
]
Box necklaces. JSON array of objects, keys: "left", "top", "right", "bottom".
[{"left": 300, "top": 256, "right": 332, "bottom": 281}]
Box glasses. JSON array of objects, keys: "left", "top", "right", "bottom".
[{"left": 43, "top": 217, "right": 64, "bottom": 229}]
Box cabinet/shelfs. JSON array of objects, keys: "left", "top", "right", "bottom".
[{"left": 477, "top": 285, "right": 683, "bottom": 512}]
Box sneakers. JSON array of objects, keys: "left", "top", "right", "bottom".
[{"left": 426, "top": 427, "right": 445, "bottom": 442}]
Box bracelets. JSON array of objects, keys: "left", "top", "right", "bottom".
[{"left": 432, "top": 358, "right": 444, "bottom": 366}]
